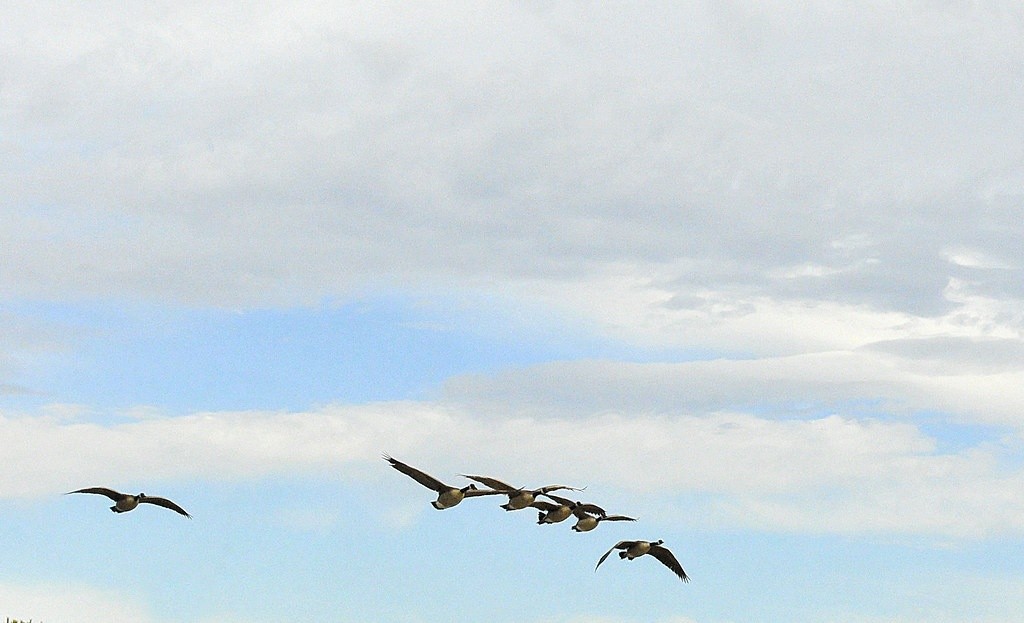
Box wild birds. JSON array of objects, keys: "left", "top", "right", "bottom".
[
  {"left": 379, "top": 448, "right": 693, "bottom": 585},
  {"left": 62, "top": 487, "right": 194, "bottom": 521}
]
